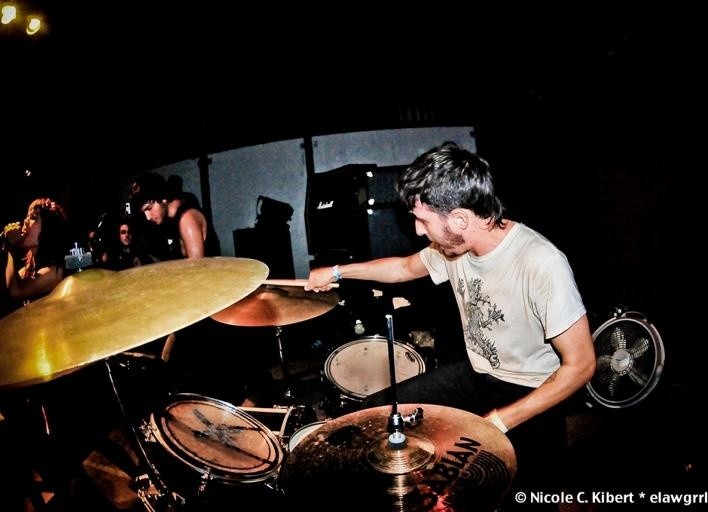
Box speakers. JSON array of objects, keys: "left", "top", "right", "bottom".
[{"left": 305, "top": 163, "right": 446, "bottom": 338}]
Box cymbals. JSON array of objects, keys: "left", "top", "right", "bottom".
[
  {"left": 0, "top": 255, "right": 269, "bottom": 390},
  {"left": 277, "top": 403, "right": 518, "bottom": 512},
  {"left": 208, "top": 285, "right": 339, "bottom": 328}
]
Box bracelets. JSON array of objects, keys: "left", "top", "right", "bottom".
[
  {"left": 333, "top": 264, "right": 343, "bottom": 282},
  {"left": 489, "top": 409, "right": 508, "bottom": 434}
]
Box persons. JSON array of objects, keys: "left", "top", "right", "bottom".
[
  {"left": 100, "top": 223, "right": 157, "bottom": 266},
  {"left": 303, "top": 148, "right": 598, "bottom": 435},
  {"left": 1, "top": 197, "right": 72, "bottom": 305},
  {"left": 137, "top": 183, "right": 222, "bottom": 259}
]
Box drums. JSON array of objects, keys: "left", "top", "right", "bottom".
[
  {"left": 286, "top": 421, "right": 327, "bottom": 453},
  {"left": 149, "top": 391, "right": 284, "bottom": 510},
  {"left": 324, "top": 335, "right": 427, "bottom": 416}
]
